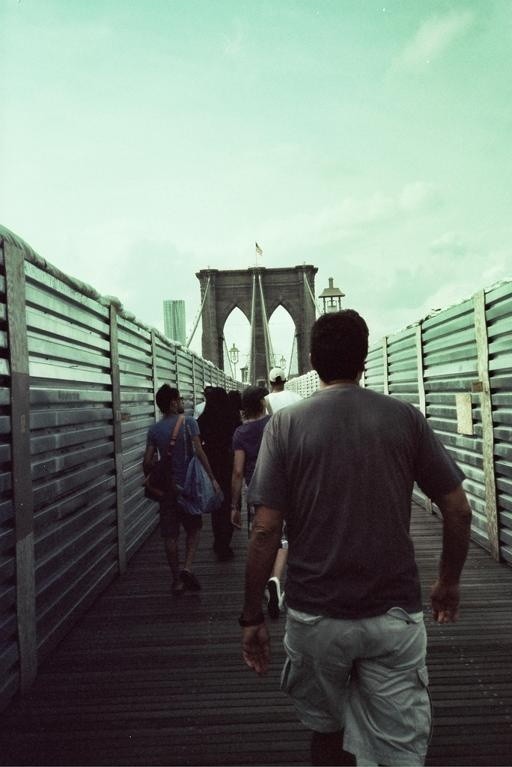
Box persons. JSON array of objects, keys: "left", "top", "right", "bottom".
[
  {"left": 239, "top": 308, "right": 472, "bottom": 767},
  {"left": 193, "top": 385, "right": 270, "bottom": 563},
  {"left": 143, "top": 383, "right": 217, "bottom": 590},
  {"left": 264, "top": 368, "right": 303, "bottom": 416},
  {"left": 230, "top": 385, "right": 289, "bottom": 620}
]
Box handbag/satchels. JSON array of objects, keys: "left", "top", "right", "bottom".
[
  {"left": 143, "top": 461, "right": 168, "bottom": 499},
  {"left": 177, "top": 455, "right": 224, "bottom": 515}
]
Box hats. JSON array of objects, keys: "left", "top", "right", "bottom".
[{"left": 269, "top": 368, "right": 286, "bottom": 382}]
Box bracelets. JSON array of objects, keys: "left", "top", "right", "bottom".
[
  {"left": 231, "top": 503, "right": 240, "bottom": 509},
  {"left": 238, "top": 615, "right": 264, "bottom": 627}
]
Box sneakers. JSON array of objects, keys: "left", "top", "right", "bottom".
[
  {"left": 172, "top": 570, "right": 200, "bottom": 594},
  {"left": 265, "top": 576, "right": 283, "bottom": 620},
  {"left": 213, "top": 541, "right": 235, "bottom": 561}
]
